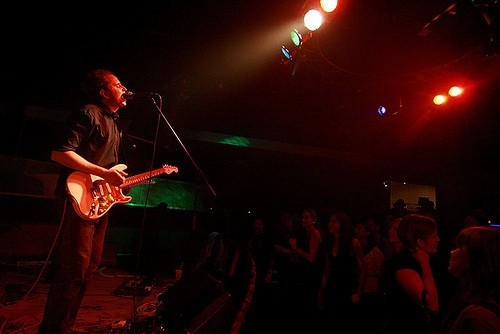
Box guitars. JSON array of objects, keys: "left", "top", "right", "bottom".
[{"left": 65, "top": 165, "right": 179, "bottom": 221}]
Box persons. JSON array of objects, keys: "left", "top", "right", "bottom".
[
  {"left": 38, "top": 67, "right": 128, "bottom": 334},
  {"left": 228, "top": 199, "right": 500, "bottom": 334},
  {"left": 378, "top": 214, "right": 440, "bottom": 334},
  {"left": 438, "top": 225, "right": 500, "bottom": 334}
]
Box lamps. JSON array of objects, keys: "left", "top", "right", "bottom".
[{"left": 280, "top": 24, "right": 312, "bottom": 62}]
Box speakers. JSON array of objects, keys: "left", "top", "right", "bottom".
[{"left": 164, "top": 269, "right": 238, "bottom": 334}]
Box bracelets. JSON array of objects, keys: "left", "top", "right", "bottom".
[{"left": 294, "top": 247, "right": 298, "bottom": 252}]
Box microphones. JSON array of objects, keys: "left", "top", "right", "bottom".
[{"left": 124, "top": 91, "right": 158, "bottom": 100}]
